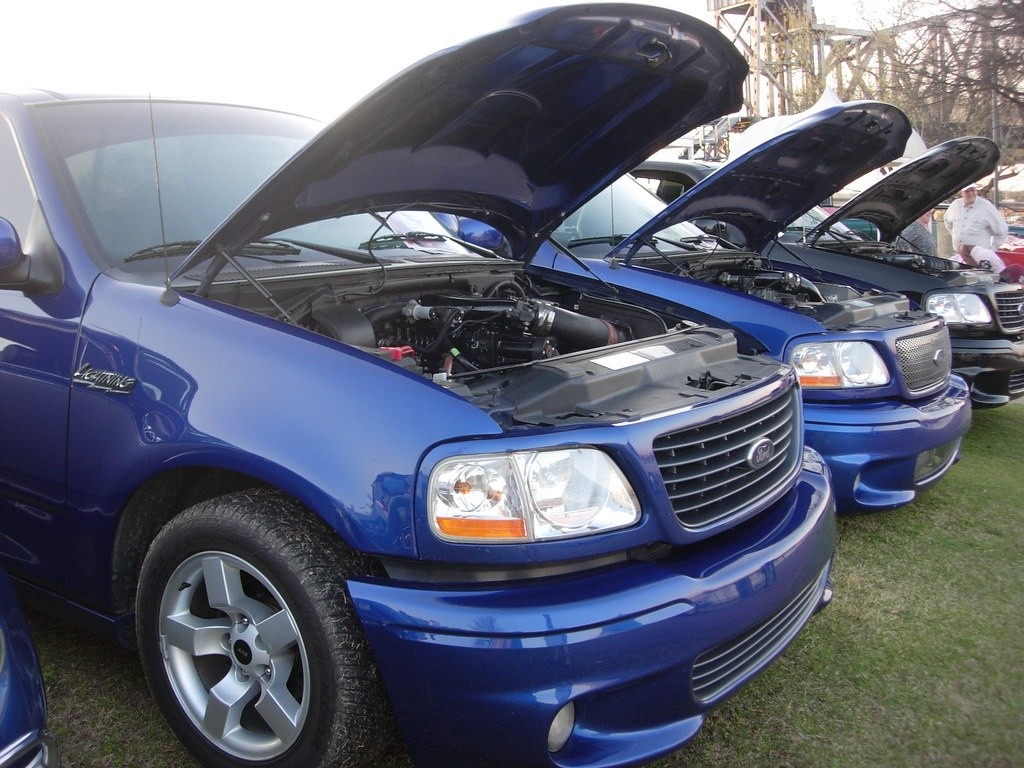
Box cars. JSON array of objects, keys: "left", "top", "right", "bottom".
[
  {"left": 1, "top": 4, "right": 844, "bottom": 768},
  {"left": 460, "top": 101, "right": 1022, "bottom": 513}
]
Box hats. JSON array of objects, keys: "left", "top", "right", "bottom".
[
  {"left": 961, "top": 183, "right": 977, "bottom": 191},
  {"left": 1007, "top": 264, "right": 1024, "bottom": 283}
]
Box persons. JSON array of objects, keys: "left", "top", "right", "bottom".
[
  {"left": 948, "top": 244, "right": 1024, "bottom": 284},
  {"left": 896, "top": 209, "right": 938, "bottom": 258},
  {"left": 943, "top": 183, "right": 1008, "bottom": 255}
]
